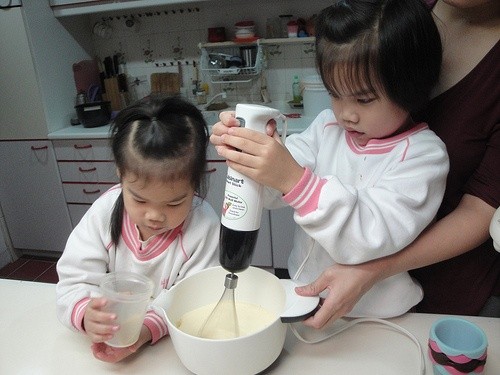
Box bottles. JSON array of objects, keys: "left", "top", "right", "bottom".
[
  {"left": 292, "top": 76, "right": 300, "bottom": 103},
  {"left": 77, "top": 89, "right": 87, "bottom": 105},
  {"left": 267, "top": 14, "right": 293, "bottom": 38}
]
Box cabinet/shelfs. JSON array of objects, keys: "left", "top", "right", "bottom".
[{"left": 0, "top": 140, "right": 296, "bottom": 276}]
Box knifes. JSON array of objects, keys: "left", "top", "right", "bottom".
[{"left": 98, "top": 54, "right": 126, "bottom": 94}]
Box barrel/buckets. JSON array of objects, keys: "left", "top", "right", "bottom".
[{"left": 299, "top": 75, "right": 334, "bottom": 120}]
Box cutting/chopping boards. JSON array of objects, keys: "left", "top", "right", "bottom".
[{"left": 150, "top": 72, "right": 180, "bottom": 94}]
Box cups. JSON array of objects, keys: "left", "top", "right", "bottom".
[
  {"left": 239, "top": 46, "right": 256, "bottom": 67},
  {"left": 97, "top": 271, "right": 154, "bottom": 349},
  {"left": 427, "top": 318, "right": 488, "bottom": 375},
  {"left": 286, "top": 20, "right": 297, "bottom": 38}
]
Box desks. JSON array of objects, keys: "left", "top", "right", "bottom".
[{"left": 0, "top": 278, "right": 500, "bottom": 375}]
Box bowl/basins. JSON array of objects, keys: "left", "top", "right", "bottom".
[
  {"left": 75, "top": 101, "right": 112, "bottom": 128},
  {"left": 232, "top": 25, "right": 256, "bottom": 39},
  {"left": 150, "top": 264, "right": 321, "bottom": 375}
]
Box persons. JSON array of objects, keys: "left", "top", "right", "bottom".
[
  {"left": 210, "top": 0, "right": 450, "bottom": 318},
  {"left": 56, "top": 91, "right": 220, "bottom": 362},
  {"left": 295, "top": 0, "right": 500, "bottom": 329}
]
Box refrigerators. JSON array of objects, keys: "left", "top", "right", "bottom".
[{"left": 0, "top": 0, "right": 97, "bottom": 260}]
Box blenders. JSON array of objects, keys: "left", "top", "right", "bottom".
[{"left": 196, "top": 102, "right": 286, "bottom": 340}]
